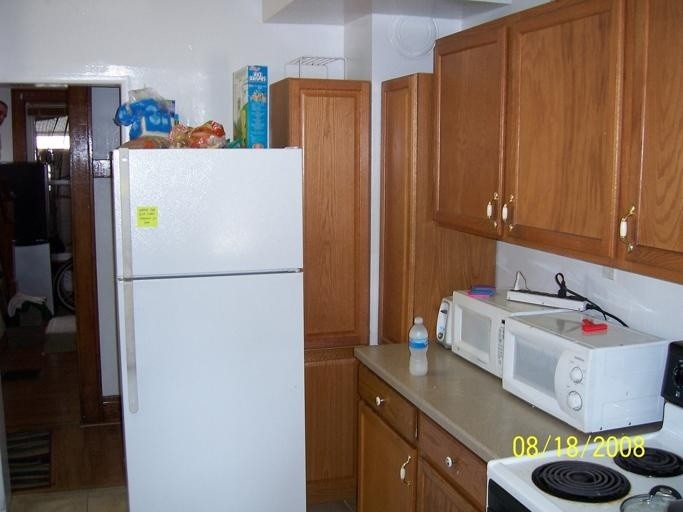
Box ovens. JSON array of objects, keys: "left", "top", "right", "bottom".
[{"left": 501, "top": 311, "right": 672, "bottom": 436}]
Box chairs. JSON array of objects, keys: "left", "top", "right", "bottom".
[{"left": 0, "top": 268, "right": 52, "bottom": 381}]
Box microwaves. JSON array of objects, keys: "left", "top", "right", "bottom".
[{"left": 450, "top": 288, "right": 555, "bottom": 379}]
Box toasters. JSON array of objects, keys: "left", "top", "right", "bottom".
[{"left": 433, "top": 295, "right": 454, "bottom": 349}]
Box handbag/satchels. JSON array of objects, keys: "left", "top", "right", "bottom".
[{"left": 3, "top": 300, "right": 51, "bottom": 326}]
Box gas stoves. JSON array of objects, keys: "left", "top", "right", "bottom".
[{"left": 490, "top": 445, "right": 682, "bottom": 511}]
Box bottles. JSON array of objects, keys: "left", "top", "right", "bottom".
[{"left": 408, "top": 317, "right": 430, "bottom": 375}]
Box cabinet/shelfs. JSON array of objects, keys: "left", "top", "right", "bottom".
[
  {"left": 621, "top": 1, "right": 683, "bottom": 284},
  {"left": 378, "top": 75, "right": 497, "bottom": 353},
  {"left": 356, "top": 361, "right": 488, "bottom": 511},
  {"left": 430, "top": 0, "right": 622, "bottom": 267},
  {"left": 269, "top": 77, "right": 371, "bottom": 509}
]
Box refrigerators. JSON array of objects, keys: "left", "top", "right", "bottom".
[{"left": 112, "top": 147, "right": 307, "bottom": 512}]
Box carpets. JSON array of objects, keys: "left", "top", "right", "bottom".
[{"left": 7, "top": 431, "right": 52, "bottom": 492}]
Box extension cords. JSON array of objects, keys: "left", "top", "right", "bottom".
[{"left": 507, "top": 289, "right": 588, "bottom": 312}]
